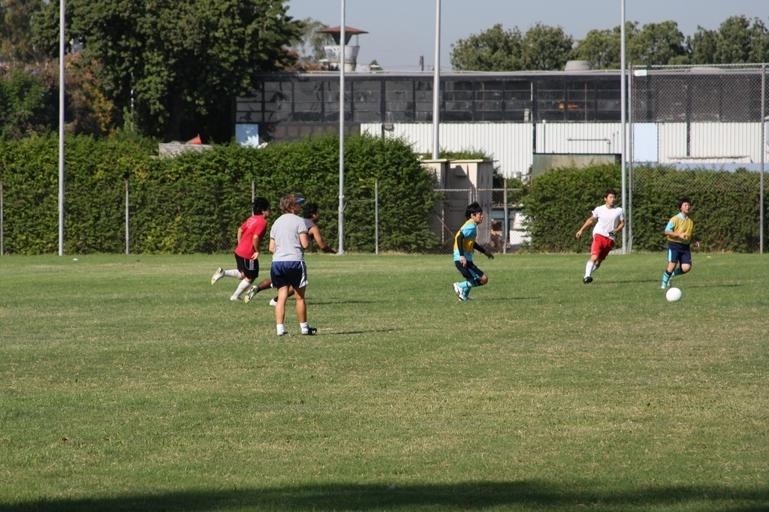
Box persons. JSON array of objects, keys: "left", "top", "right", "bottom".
[
  {"left": 268, "top": 194, "right": 319, "bottom": 336},
  {"left": 660, "top": 198, "right": 695, "bottom": 289},
  {"left": 451, "top": 201, "right": 496, "bottom": 301},
  {"left": 241, "top": 201, "right": 340, "bottom": 304},
  {"left": 574, "top": 189, "right": 626, "bottom": 286},
  {"left": 209, "top": 195, "right": 271, "bottom": 302}
]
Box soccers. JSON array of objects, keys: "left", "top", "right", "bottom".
[{"left": 666, "top": 288, "right": 682, "bottom": 302}]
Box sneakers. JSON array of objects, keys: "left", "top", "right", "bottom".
[
  {"left": 661, "top": 279, "right": 670, "bottom": 289},
  {"left": 245, "top": 286, "right": 259, "bottom": 304},
  {"left": 211, "top": 266, "right": 225, "bottom": 285},
  {"left": 453, "top": 282, "right": 469, "bottom": 301},
  {"left": 269, "top": 298, "right": 277, "bottom": 306},
  {"left": 276, "top": 328, "right": 288, "bottom": 336},
  {"left": 583, "top": 275, "right": 593, "bottom": 283},
  {"left": 230, "top": 296, "right": 243, "bottom": 302},
  {"left": 302, "top": 325, "right": 317, "bottom": 335}
]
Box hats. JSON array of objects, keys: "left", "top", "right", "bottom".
[{"left": 295, "top": 196, "right": 306, "bottom": 203}]
